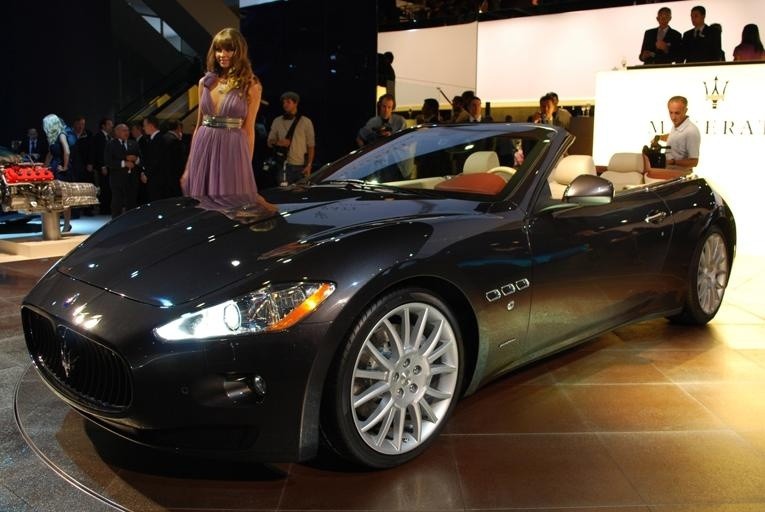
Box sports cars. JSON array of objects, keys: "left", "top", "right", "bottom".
[{"left": 15, "top": 123, "right": 744, "bottom": 479}]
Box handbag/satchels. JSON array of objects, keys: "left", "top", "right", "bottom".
[{"left": 253, "top": 154, "right": 284, "bottom": 184}]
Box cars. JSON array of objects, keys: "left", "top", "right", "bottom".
[{"left": 1, "top": 146, "right": 43, "bottom": 229}]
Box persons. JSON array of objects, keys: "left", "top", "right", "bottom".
[
  {"left": 19, "top": 114, "right": 196, "bottom": 235},
  {"left": 185, "top": 194, "right": 279, "bottom": 234},
  {"left": 179, "top": 25, "right": 264, "bottom": 195},
  {"left": 650, "top": 96, "right": 701, "bottom": 174},
  {"left": 252, "top": 120, "right": 269, "bottom": 190},
  {"left": 732, "top": 23, "right": 764, "bottom": 60},
  {"left": 638, "top": 6, "right": 682, "bottom": 64},
  {"left": 355, "top": 84, "right": 574, "bottom": 185},
  {"left": 676, "top": 5, "right": 722, "bottom": 63},
  {"left": 700, "top": 23, "right": 726, "bottom": 64},
  {"left": 266, "top": 89, "right": 316, "bottom": 185}
]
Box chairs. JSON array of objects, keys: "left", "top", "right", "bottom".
[
  {"left": 461, "top": 150, "right": 500, "bottom": 175},
  {"left": 547, "top": 153, "right": 595, "bottom": 200},
  {"left": 597, "top": 150, "right": 652, "bottom": 194}
]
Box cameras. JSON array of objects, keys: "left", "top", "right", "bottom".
[{"left": 540, "top": 113, "right": 547, "bottom": 118}]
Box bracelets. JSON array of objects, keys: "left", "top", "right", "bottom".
[
  {"left": 672, "top": 159, "right": 676, "bottom": 166},
  {"left": 308, "top": 163, "right": 314, "bottom": 167},
  {"left": 655, "top": 135, "right": 660, "bottom": 142}
]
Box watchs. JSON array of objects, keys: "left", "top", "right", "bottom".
[{"left": 376, "top": 50, "right": 397, "bottom": 87}]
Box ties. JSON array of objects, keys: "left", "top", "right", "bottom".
[
  {"left": 696, "top": 28, "right": 701, "bottom": 39},
  {"left": 121, "top": 139, "right": 126, "bottom": 151}
]
